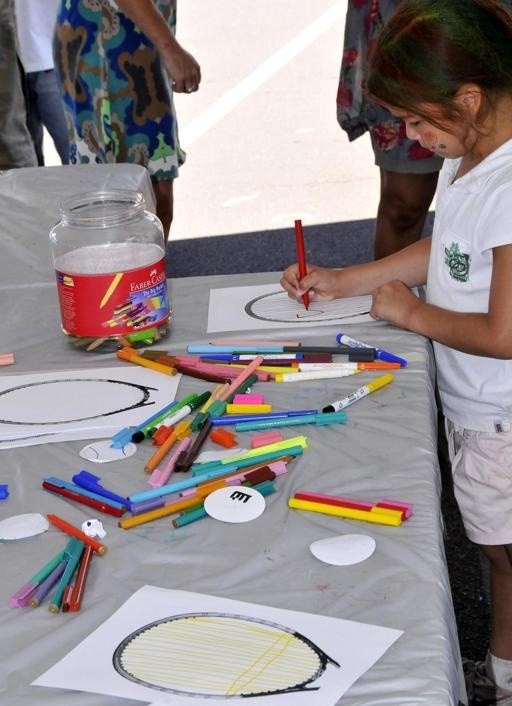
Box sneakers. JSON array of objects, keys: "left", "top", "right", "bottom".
[{"left": 462, "top": 654, "right": 511, "bottom": 705}]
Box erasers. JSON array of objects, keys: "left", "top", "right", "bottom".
[{"left": 0, "top": 353, "right": 15, "bottom": 365}]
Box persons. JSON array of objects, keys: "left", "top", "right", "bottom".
[
  {"left": 0, "top": 0, "right": 38, "bottom": 176},
  {"left": 51, "top": 0, "right": 202, "bottom": 244},
  {"left": 337, "top": 0, "right": 512, "bottom": 260},
  {"left": 14, "top": 0, "right": 69, "bottom": 167},
  {"left": 281, "top": 0, "right": 512, "bottom": 705}
]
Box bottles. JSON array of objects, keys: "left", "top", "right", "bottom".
[{"left": 49, "top": 189, "right": 174, "bottom": 354}]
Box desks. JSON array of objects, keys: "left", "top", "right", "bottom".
[{"left": 1, "top": 269, "right": 467, "bottom": 706}]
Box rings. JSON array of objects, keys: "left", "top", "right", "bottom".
[{"left": 185, "top": 85, "right": 195, "bottom": 91}]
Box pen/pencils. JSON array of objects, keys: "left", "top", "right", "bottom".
[
  {"left": 99, "top": 273, "right": 148, "bottom": 327},
  {"left": 287, "top": 490, "right": 413, "bottom": 526},
  {"left": 0, "top": 485, "right": 8, "bottom": 498},
  {"left": 295, "top": 220, "right": 310, "bottom": 310},
  {"left": 74, "top": 338, "right": 104, "bottom": 351},
  {"left": 9, "top": 514, "right": 106, "bottom": 613},
  {"left": 42, "top": 333, "right": 407, "bottom": 529}
]
